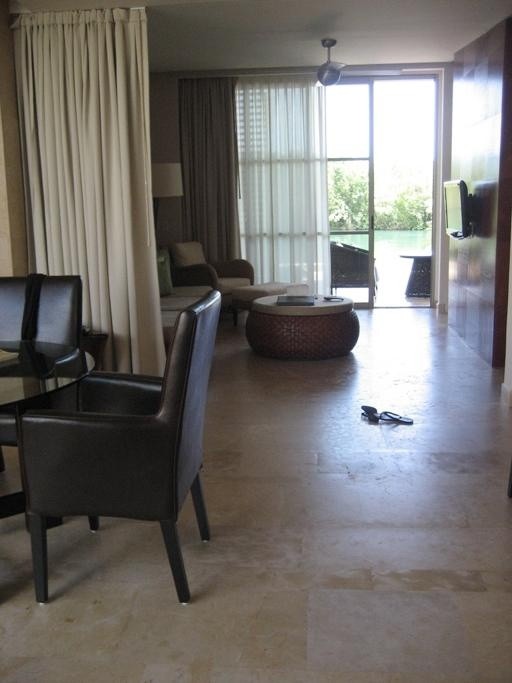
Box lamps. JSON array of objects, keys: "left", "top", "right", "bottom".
[{"left": 151, "top": 163, "right": 185, "bottom": 227}]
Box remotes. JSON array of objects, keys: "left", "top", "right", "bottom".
[{"left": 324, "top": 297, "right": 344, "bottom": 300}]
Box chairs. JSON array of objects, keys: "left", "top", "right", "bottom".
[
  {"left": 24, "top": 290, "right": 222, "bottom": 602},
  {"left": 0, "top": 276, "right": 84, "bottom": 446},
  {"left": 168, "top": 239, "right": 256, "bottom": 326},
  {"left": 330, "top": 241, "right": 378, "bottom": 302}
]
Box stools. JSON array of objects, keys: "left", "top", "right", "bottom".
[{"left": 232, "top": 282, "right": 306, "bottom": 328}]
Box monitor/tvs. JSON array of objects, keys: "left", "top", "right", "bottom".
[{"left": 444, "top": 180, "right": 474, "bottom": 240}]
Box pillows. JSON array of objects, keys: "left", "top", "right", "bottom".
[{"left": 157, "top": 248, "right": 172, "bottom": 296}]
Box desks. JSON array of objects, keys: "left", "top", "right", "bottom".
[
  {"left": 0, "top": 340, "right": 96, "bottom": 529},
  {"left": 399, "top": 255, "right": 434, "bottom": 298}
]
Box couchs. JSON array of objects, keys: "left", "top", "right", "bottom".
[{"left": 160, "top": 286, "right": 213, "bottom": 353}]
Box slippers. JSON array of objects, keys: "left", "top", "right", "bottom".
[
  {"left": 374, "top": 412, "right": 413, "bottom": 425},
  {"left": 361, "top": 406, "right": 378, "bottom": 422}
]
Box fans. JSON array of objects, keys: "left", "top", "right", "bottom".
[{"left": 312, "top": 39, "right": 348, "bottom": 88}]
